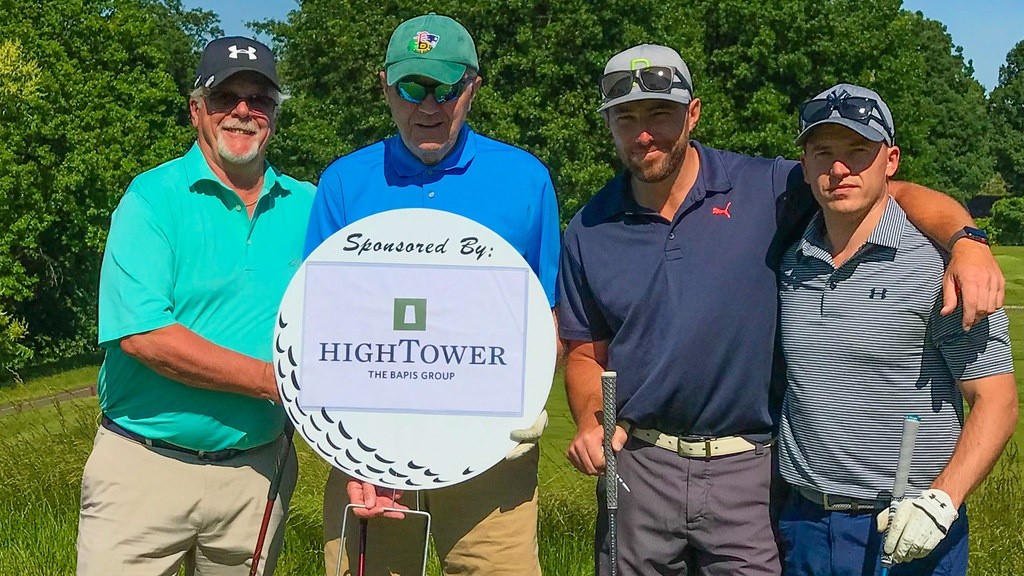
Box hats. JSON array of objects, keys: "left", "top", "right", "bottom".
[
  {"left": 596, "top": 44, "right": 693, "bottom": 113},
  {"left": 383, "top": 12, "right": 480, "bottom": 85},
  {"left": 193, "top": 36, "right": 283, "bottom": 93},
  {"left": 794, "top": 83, "right": 895, "bottom": 148}
]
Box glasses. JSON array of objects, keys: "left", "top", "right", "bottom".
[
  {"left": 201, "top": 89, "right": 279, "bottom": 115},
  {"left": 799, "top": 97, "right": 893, "bottom": 147},
  {"left": 395, "top": 77, "right": 475, "bottom": 104},
  {"left": 599, "top": 66, "right": 694, "bottom": 102}
]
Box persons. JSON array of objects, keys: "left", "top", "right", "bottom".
[
  {"left": 304, "top": 15, "right": 562, "bottom": 576},
  {"left": 560, "top": 42, "right": 1005, "bottom": 576},
  {"left": 774, "top": 83, "right": 1019, "bottom": 576},
  {"left": 75, "top": 35, "right": 320, "bottom": 576}
]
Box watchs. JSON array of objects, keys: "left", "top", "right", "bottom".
[{"left": 946, "top": 226, "right": 992, "bottom": 253}]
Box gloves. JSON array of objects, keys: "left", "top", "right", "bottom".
[
  {"left": 505, "top": 408, "right": 549, "bottom": 461},
  {"left": 876, "top": 489, "right": 960, "bottom": 563}
]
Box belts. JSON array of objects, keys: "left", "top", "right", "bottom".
[
  {"left": 788, "top": 482, "right": 891, "bottom": 511},
  {"left": 616, "top": 418, "right": 778, "bottom": 457},
  {"left": 101, "top": 411, "right": 286, "bottom": 462}
]
